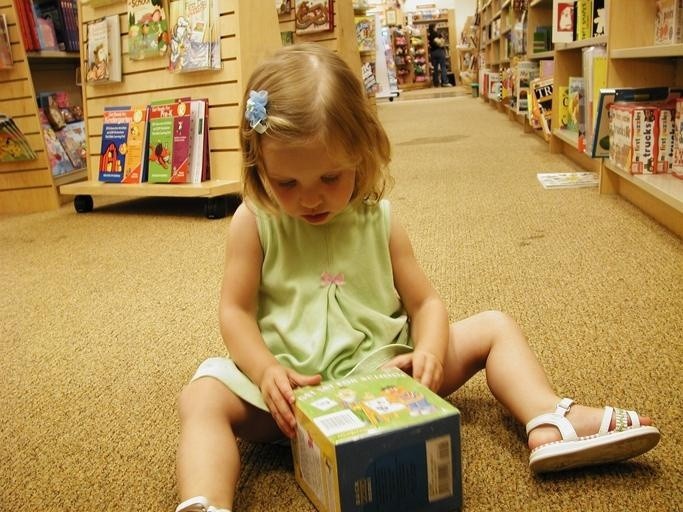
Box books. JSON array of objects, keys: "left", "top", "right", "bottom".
[{"left": 1, "top": 0, "right": 683, "bottom": 189}]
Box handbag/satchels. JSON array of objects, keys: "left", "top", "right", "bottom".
[{"left": 434, "top": 37, "right": 445, "bottom": 47}]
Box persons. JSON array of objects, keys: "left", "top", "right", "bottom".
[{"left": 174, "top": 39, "right": 661, "bottom": 512}]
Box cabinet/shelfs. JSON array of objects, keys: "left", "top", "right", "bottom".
[
  {"left": 0, "top": 0, "right": 88, "bottom": 217},
  {"left": 393, "top": 10, "right": 459, "bottom": 91}
]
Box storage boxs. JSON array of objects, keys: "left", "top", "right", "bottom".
[{"left": 289, "top": 366, "right": 463, "bottom": 512}]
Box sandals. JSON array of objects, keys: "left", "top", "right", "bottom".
[{"left": 525, "top": 397, "right": 660, "bottom": 472}]
